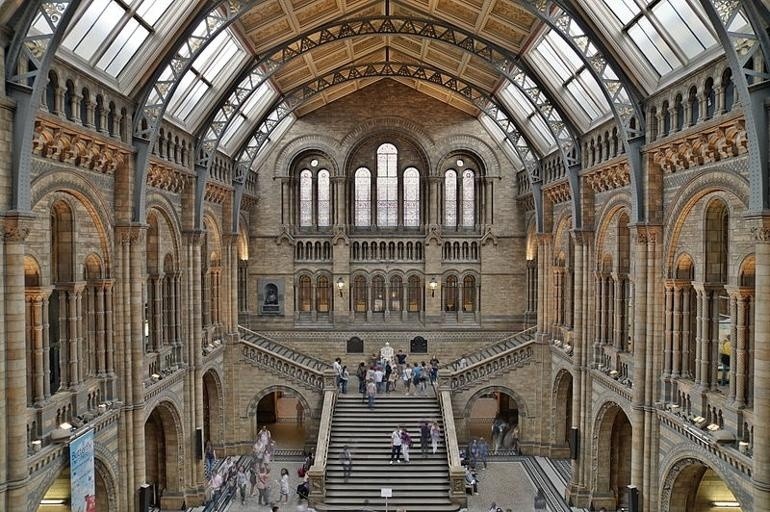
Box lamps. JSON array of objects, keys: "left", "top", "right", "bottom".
[
  {"left": 428, "top": 278, "right": 437, "bottom": 298},
  {"left": 335, "top": 276, "right": 345, "bottom": 298}
]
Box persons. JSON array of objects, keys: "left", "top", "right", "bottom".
[
  {"left": 458, "top": 438, "right": 488, "bottom": 492},
  {"left": 390, "top": 425, "right": 413, "bottom": 464},
  {"left": 334, "top": 350, "right": 440, "bottom": 408},
  {"left": 491, "top": 408, "right": 520, "bottom": 456},
  {"left": 460, "top": 354, "right": 467, "bottom": 384},
  {"left": 340, "top": 445, "right": 352, "bottom": 483},
  {"left": 296, "top": 400, "right": 305, "bottom": 428},
  {"left": 421, "top": 421, "right": 440, "bottom": 458},
  {"left": 488, "top": 501, "right": 512, "bottom": 511},
  {"left": 534, "top": 488, "right": 548, "bottom": 512},
  {"left": 720, "top": 335, "right": 730, "bottom": 385},
  {"left": 599, "top": 507, "right": 607, "bottom": 512},
  {"left": 200, "top": 426, "right": 313, "bottom": 512}
]
